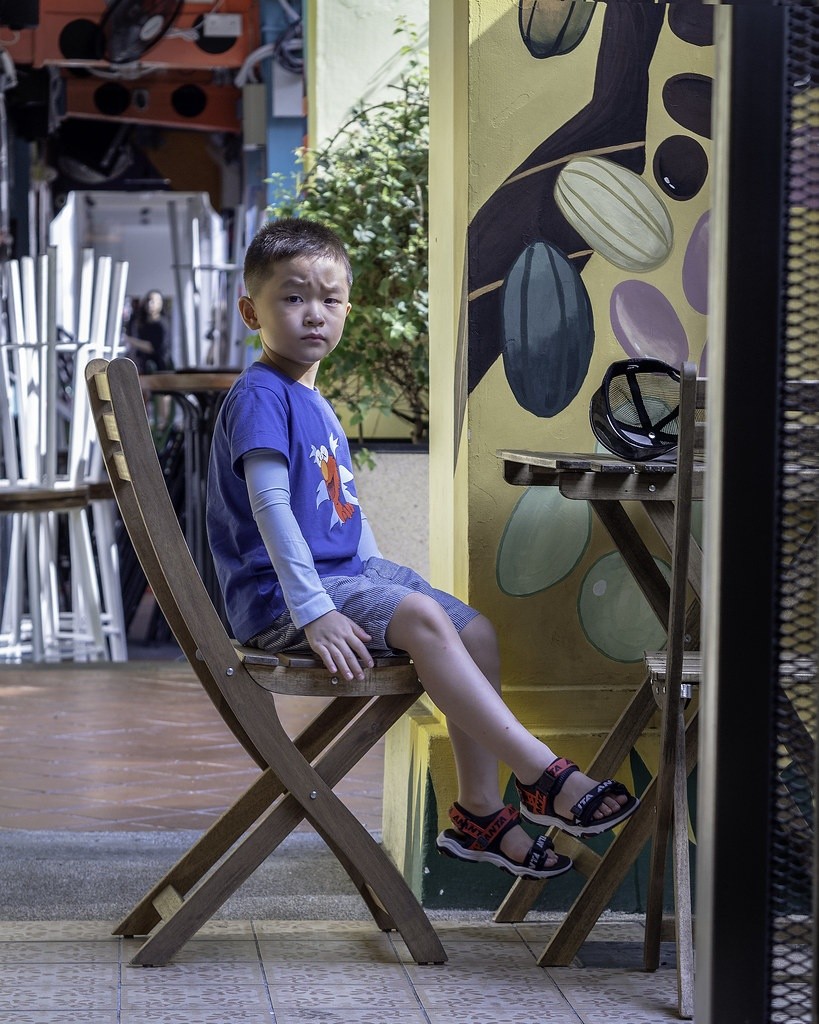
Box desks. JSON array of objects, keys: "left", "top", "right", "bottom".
[
  {"left": 132, "top": 366, "right": 244, "bottom": 641},
  {"left": 493, "top": 446, "right": 819, "bottom": 966}
]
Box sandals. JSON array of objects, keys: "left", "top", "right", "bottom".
[
  {"left": 434, "top": 801, "right": 572, "bottom": 879},
  {"left": 513, "top": 756, "right": 639, "bottom": 837}
]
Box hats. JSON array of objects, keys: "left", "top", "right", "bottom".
[{"left": 589, "top": 356, "right": 702, "bottom": 459}]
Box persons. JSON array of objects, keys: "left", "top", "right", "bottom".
[
  {"left": 205, "top": 220, "right": 639, "bottom": 881},
  {"left": 123, "top": 290, "right": 177, "bottom": 429}
]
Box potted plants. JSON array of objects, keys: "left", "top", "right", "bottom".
[{"left": 245, "top": 16, "right": 429, "bottom": 584}]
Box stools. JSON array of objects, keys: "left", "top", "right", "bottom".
[{"left": 0, "top": 245, "right": 131, "bottom": 664}]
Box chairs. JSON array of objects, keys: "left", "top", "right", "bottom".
[
  {"left": 85, "top": 358, "right": 450, "bottom": 967},
  {"left": 642, "top": 362, "right": 819, "bottom": 1018}
]
